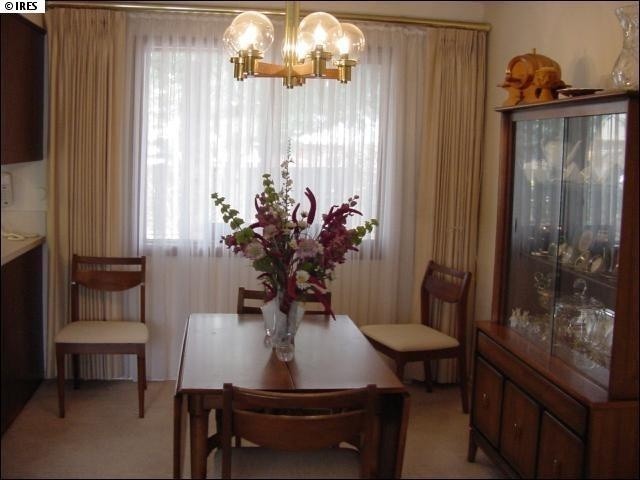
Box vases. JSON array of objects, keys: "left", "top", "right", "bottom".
[
  {"left": 612, "top": 4, "right": 639, "bottom": 92},
  {"left": 256, "top": 296, "right": 305, "bottom": 362}
]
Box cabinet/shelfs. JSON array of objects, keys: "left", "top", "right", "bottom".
[
  {"left": 3, "top": 240, "right": 49, "bottom": 432},
  {"left": 466, "top": 320, "right": 640, "bottom": 479},
  {"left": 489, "top": 88, "right": 640, "bottom": 401},
  {"left": 1, "top": 13, "right": 45, "bottom": 166}
]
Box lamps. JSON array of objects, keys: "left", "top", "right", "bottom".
[{"left": 221, "top": 1, "right": 367, "bottom": 88}]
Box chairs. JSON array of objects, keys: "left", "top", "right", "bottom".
[
  {"left": 233, "top": 286, "right": 334, "bottom": 321},
  {"left": 361, "top": 259, "right": 472, "bottom": 415},
  {"left": 52, "top": 253, "right": 154, "bottom": 420},
  {"left": 212, "top": 382, "right": 377, "bottom": 474}
]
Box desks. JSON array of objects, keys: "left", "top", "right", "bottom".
[{"left": 173, "top": 313, "right": 412, "bottom": 475}]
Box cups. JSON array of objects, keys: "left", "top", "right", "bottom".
[{"left": 276, "top": 327, "right": 294, "bottom": 361}]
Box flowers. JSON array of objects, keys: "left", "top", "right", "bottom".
[{"left": 210, "top": 138, "right": 379, "bottom": 330}]
